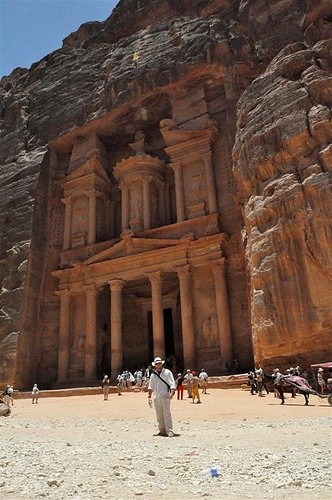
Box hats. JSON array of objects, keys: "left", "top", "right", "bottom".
[
  {"left": 192, "top": 370, "right": 197, "bottom": 374},
  {"left": 187, "top": 369, "right": 190, "bottom": 371},
  {"left": 152, "top": 357, "right": 165, "bottom": 366},
  {"left": 104, "top": 375, "right": 108, "bottom": 378},
  {"left": 201, "top": 368, "right": 206, "bottom": 371},
  {"left": 178, "top": 373, "right": 181, "bottom": 376},
  {"left": 318, "top": 368, "right": 324, "bottom": 372}
]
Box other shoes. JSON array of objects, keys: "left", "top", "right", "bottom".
[
  {"left": 153, "top": 431, "right": 168, "bottom": 437},
  {"left": 168, "top": 431, "right": 174, "bottom": 437}
]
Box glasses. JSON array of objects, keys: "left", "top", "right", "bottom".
[{"left": 155, "top": 363, "right": 162, "bottom": 365}]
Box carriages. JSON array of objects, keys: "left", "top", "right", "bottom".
[{"left": 254, "top": 361, "right": 332, "bottom": 407}]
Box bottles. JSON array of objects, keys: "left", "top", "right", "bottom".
[{"left": 149, "top": 400, "right": 153, "bottom": 408}]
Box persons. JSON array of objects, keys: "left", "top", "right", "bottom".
[
  {"left": 32, "top": 384, "right": 39, "bottom": 404},
  {"left": 6, "top": 385, "right": 13, "bottom": 408},
  {"left": 117, "top": 375, "right": 122, "bottom": 396},
  {"left": 190, "top": 371, "right": 201, "bottom": 403},
  {"left": 249, "top": 366, "right": 308, "bottom": 399},
  {"left": 102, "top": 375, "right": 109, "bottom": 400},
  {"left": 137, "top": 369, "right": 142, "bottom": 387},
  {"left": 148, "top": 358, "right": 177, "bottom": 436},
  {"left": 317, "top": 368, "right": 326, "bottom": 394},
  {"left": 199, "top": 369, "right": 208, "bottom": 394},
  {"left": 184, "top": 369, "right": 193, "bottom": 398},
  {"left": 176, "top": 373, "right": 184, "bottom": 400},
  {"left": 121, "top": 370, "right": 130, "bottom": 389}
]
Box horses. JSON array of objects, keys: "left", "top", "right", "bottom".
[{"left": 261, "top": 371, "right": 311, "bottom": 405}]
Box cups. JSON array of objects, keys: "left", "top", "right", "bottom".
[{"left": 211, "top": 467, "right": 217, "bottom": 478}]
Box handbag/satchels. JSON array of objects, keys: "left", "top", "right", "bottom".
[{"left": 201, "top": 372, "right": 206, "bottom": 381}]
